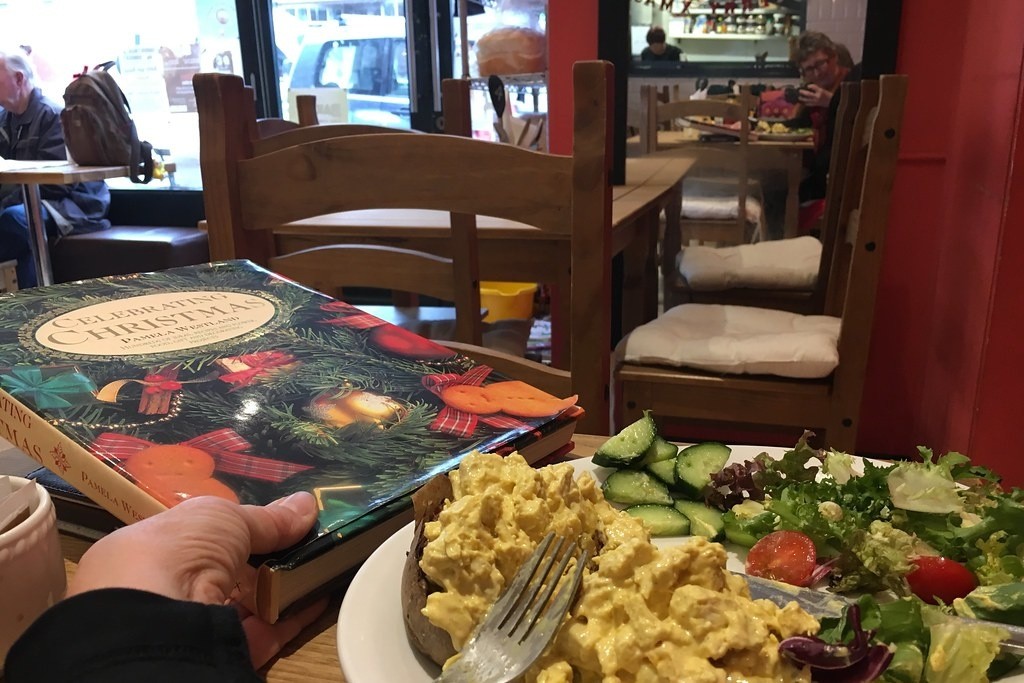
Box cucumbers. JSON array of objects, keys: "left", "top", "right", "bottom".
[{"left": 590, "top": 410, "right": 732, "bottom": 542}]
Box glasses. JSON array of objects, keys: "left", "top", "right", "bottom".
[{"left": 804, "top": 56, "right": 832, "bottom": 76}]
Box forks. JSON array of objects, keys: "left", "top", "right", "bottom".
[{"left": 436, "top": 533, "right": 589, "bottom": 683}]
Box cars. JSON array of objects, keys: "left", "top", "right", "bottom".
[{"left": 289, "top": 31, "right": 539, "bottom": 146}]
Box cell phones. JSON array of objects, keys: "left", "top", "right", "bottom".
[{"left": 784, "top": 87, "right": 814, "bottom": 103}]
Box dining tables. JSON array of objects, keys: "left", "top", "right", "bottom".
[
  {"left": 268, "top": 154, "right": 696, "bottom": 370},
  {"left": 624, "top": 124, "right": 817, "bottom": 240},
  {"left": 0, "top": 158, "right": 178, "bottom": 285}
]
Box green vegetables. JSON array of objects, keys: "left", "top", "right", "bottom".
[{"left": 708, "top": 428, "right": 1024, "bottom": 683}]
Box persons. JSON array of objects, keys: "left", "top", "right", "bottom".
[
  {"left": 830, "top": 41, "right": 857, "bottom": 71},
  {"left": 789, "top": 31, "right": 885, "bottom": 195},
  {"left": 4, "top": 490, "right": 318, "bottom": 683},
  {"left": 0, "top": 44, "right": 112, "bottom": 292},
  {"left": 641, "top": 28, "right": 684, "bottom": 61}
]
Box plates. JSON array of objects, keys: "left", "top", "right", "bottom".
[
  {"left": 335, "top": 444, "right": 1024, "bottom": 683},
  {"left": 751, "top": 129, "right": 815, "bottom": 142}
]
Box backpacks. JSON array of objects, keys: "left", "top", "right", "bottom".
[{"left": 62, "top": 62, "right": 154, "bottom": 183}]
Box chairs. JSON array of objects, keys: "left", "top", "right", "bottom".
[
  {"left": 189, "top": 57, "right": 622, "bottom": 442},
  {"left": 672, "top": 78, "right": 865, "bottom": 312},
  {"left": 605, "top": 74, "right": 910, "bottom": 452},
  {"left": 637, "top": 81, "right": 770, "bottom": 254}
]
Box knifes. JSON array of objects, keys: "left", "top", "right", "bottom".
[{"left": 723, "top": 569, "right": 1023, "bottom": 655}]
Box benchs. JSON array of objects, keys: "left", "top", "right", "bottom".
[{"left": 51, "top": 223, "right": 209, "bottom": 283}]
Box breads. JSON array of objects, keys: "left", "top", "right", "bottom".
[{"left": 476, "top": 25, "right": 548, "bottom": 75}]
